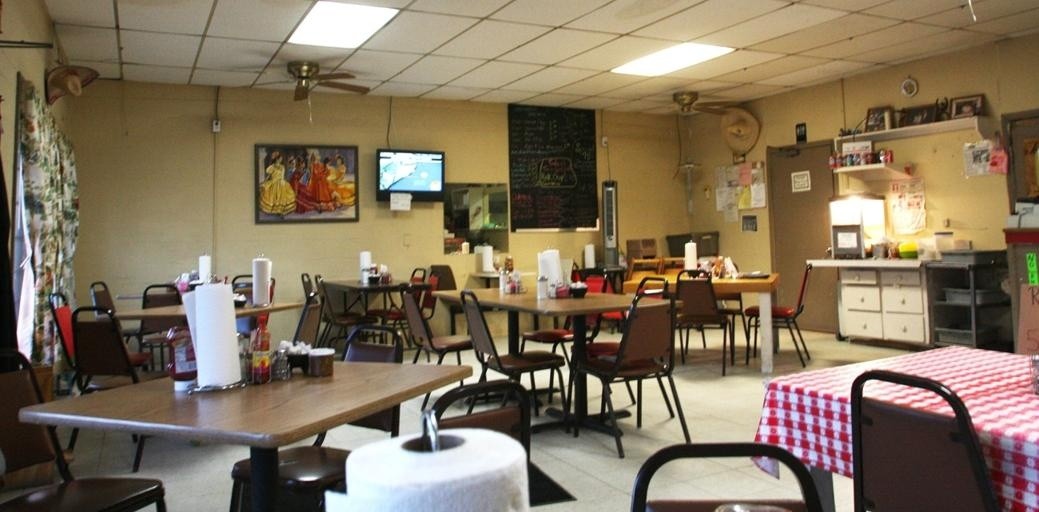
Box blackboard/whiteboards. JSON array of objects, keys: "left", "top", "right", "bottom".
[{"left": 508, "top": 103, "right": 599, "bottom": 233}]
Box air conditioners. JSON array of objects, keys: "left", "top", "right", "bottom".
[{"left": 601, "top": 179, "right": 619, "bottom": 267}]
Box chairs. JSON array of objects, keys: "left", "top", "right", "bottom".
[{"left": 850, "top": 370, "right": 999, "bottom": 510}]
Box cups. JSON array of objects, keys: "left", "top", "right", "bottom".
[{"left": 306, "top": 346, "right": 337, "bottom": 377}]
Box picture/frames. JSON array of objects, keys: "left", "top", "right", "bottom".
[
  {"left": 252, "top": 142, "right": 360, "bottom": 226},
  {"left": 1000, "top": 108, "right": 1039, "bottom": 216},
  {"left": 864, "top": 94, "right": 984, "bottom": 133}
]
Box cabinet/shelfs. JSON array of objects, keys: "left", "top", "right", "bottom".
[
  {"left": 926, "top": 261, "right": 1011, "bottom": 348},
  {"left": 805, "top": 258, "right": 941, "bottom": 350}
]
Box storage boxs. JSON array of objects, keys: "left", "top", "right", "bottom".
[
  {"left": 665, "top": 231, "right": 720, "bottom": 257},
  {"left": 934, "top": 249, "right": 1007, "bottom": 346}
]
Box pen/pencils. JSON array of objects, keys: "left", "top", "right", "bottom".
[
  {"left": 208, "top": 274, "right": 217, "bottom": 283},
  {"left": 224, "top": 276, "right": 228, "bottom": 284}
]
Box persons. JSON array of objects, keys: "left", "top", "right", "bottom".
[
  {"left": 259, "top": 151, "right": 355, "bottom": 217},
  {"left": 494, "top": 255, "right": 521, "bottom": 292},
  {"left": 471, "top": 205, "right": 482, "bottom": 229}
]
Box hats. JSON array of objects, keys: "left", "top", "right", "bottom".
[{"left": 271, "top": 151, "right": 280, "bottom": 161}]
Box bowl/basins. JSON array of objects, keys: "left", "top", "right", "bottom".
[
  {"left": 568, "top": 286, "right": 589, "bottom": 299},
  {"left": 234, "top": 299, "right": 248, "bottom": 307}
]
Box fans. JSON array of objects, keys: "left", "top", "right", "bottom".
[
  {"left": 233, "top": 60, "right": 369, "bottom": 102},
  {"left": 638, "top": 90, "right": 743, "bottom": 116}
]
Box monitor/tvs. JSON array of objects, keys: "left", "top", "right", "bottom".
[{"left": 376, "top": 148, "right": 446, "bottom": 202}]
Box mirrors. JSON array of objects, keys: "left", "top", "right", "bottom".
[{"left": 442, "top": 181, "right": 509, "bottom": 255}]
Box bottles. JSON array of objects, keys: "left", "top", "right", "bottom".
[
  {"left": 176, "top": 269, "right": 219, "bottom": 292},
  {"left": 493, "top": 254, "right": 523, "bottom": 295},
  {"left": 697, "top": 255, "right": 733, "bottom": 281},
  {"left": 827, "top": 145, "right": 893, "bottom": 171},
  {"left": 536, "top": 276, "right": 572, "bottom": 300},
  {"left": 1029, "top": 352, "right": 1039, "bottom": 394},
  {"left": 361, "top": 263, "right": 392, "bottom": 285},
  {"left": 935, "top": 231, "right": 954, "bottom": 252},
  {"left": 166, "top": 313, "right": 290, "bottom": 396}
]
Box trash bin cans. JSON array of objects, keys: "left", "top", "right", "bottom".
[{"left": 574, "top": 268, "right": 613, "bottom": 331}]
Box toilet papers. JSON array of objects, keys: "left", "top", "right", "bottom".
[
  {"left": 359, "top": 251, "right": 372, "bottom": 285},
  {"left": 584, "top": 245, "right": 595, "bottom": 269},
  {"left": 462, "top": 242, "right": 469, "bottom": 254},
  {"left": 198, "top": 255, "right": 212, "bottom": 283},
  {"left": 180, "top": 283, "right": 243, "bottom": 387},
  {"left": 685, "top": 243, "right": 697, "bottom": 270},
  {"left": 252, "top": 259, "right": 271, "bottom": 305},
  {"left": 538, "top": 250, "right": 564, "bottom": 298},
  {"left": 481, "top": 246, "right": 494, "bottom": 272},
  {"left": 345, "top": 427, "right": 530, "bottom": 512}
]
